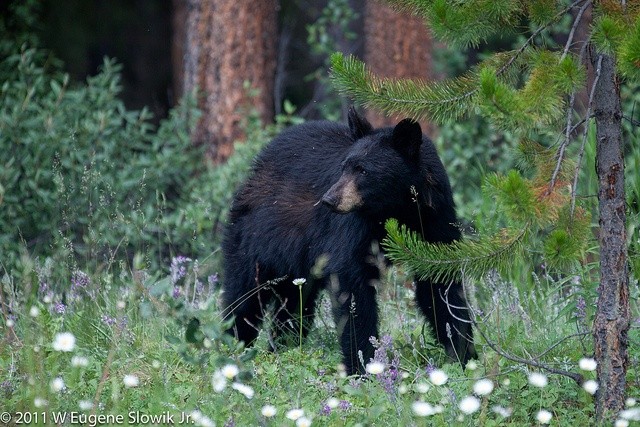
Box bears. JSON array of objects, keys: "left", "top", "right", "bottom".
[{"left": 217, "top": 102, "right": 480, "bottom": 379}]
